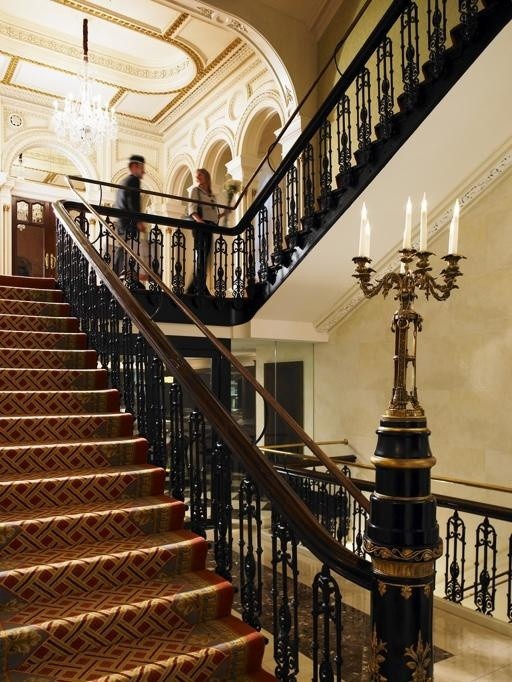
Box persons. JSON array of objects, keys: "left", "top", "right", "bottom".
[
  {"left": 186, "top": 167, "right": 228, "bottom": 299},
  {"left": 107, "top": 154, "right": 149, "bottom": 291}
]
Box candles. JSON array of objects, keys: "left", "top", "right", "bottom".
[{"left": 358, "top": 188, "right": 461, "bottom": 274}]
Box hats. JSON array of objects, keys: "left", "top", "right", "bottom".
[{"left": 128, "top": 155, "right": 143, "bottom": 165}]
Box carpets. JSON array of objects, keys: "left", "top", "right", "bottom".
[{"left": 205, "top": 542, "right": 452, "bottom": 681}]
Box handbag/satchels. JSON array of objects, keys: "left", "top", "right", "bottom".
[{"left": 181, "top": 204, "right": 202, "bottom": 222}]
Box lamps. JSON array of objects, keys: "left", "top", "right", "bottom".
[{"left": 51, "top": 19, "right": 121, "bottom": 149}]
